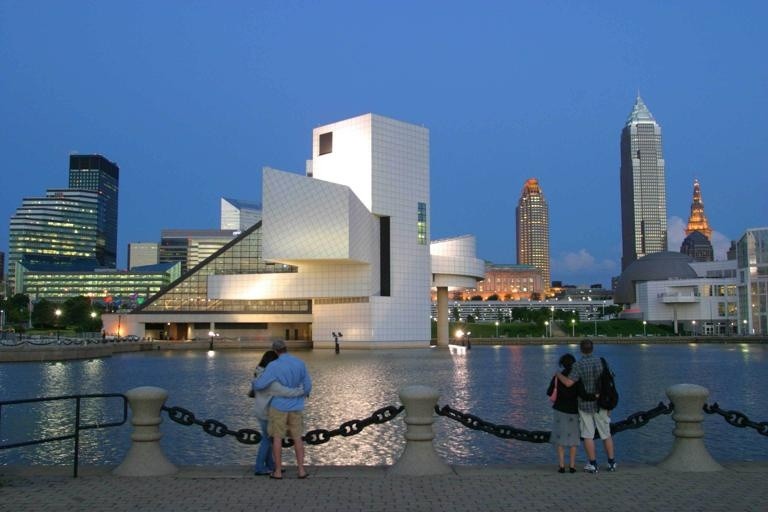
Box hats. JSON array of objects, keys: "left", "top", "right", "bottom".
[{"left": 272, "top": 338, "right": 286, "bottom": 350}]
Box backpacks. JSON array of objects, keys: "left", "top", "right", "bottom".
[{"left": 596, "top": 357, "right": 619, "bottom": 413}]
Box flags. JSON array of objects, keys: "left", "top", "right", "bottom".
[
  {"left": 120, "top": 268, "right": 128, "bottom": 274},
  {"left": 127, "top": 292, "right": 137, "bottom": 300},
  {"left": 101, "top": 295, "right": 112, "bottom": 303},
  {"left": 112, "top": 295, "right": 121, "bottom": 305}
]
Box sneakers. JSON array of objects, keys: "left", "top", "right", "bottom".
[
  {"left": 255, "top": 468, "right": 309, "bottom": 480},
  {"left": 559, "top": 462, "right": 617, "bottom": 473}
]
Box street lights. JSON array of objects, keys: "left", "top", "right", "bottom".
[
  {"left": 466, "top": 332, "right": 471, "bottom": 350},
  {"left": 20, "top": 309, "right": 23, "bottom": 342},
  {"left": 495, "top": 321, "right": 500, "bottom": 338},
  {"left": 208, "top": 332, "right": 215, "bottom": 350},
  {"left": 743, "top": 319, "right": 748, "bottom": 337},
  {"left": 91, "top": 312, "right": 97, "bottom": 339},
  {"left": 455, "top": 330, "right": 465, "bottom": 346},
  {"left": 332, "top": 332, "right": 343, "bottom": 354},
  {"left": 55, "top": 309, "right": 62, "bottom": 341},
  {"left": 691, "top": 321, "right": 696, "bottom": 337},
  {"left": 643, "top": 320, "right": 647, "bottom": 336},
  {"left": 544, "top": 307, "right": 554, "bottom": 337},
  {"left": 0, "top": 309, "right": 4, "bottom": 331},
  {"left": 571, "top": 319, "right": 575, "bottom": 337}
]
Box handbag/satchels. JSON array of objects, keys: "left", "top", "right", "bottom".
[{"left": 550, "top": 388, "right": 558, "bottom": 402}]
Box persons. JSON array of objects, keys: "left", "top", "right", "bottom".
[
  {"left": 552, "top": 338, "right": 616, "bottom": 475},
  {"left": 545, "top": 353, "right": 601, "bottom": 475},
  {"left": 245, "top": 350, "right": 305, "bottom": 476},
  {"left": 249, "top": 338, "right": 314, "bottom": 481}
]
